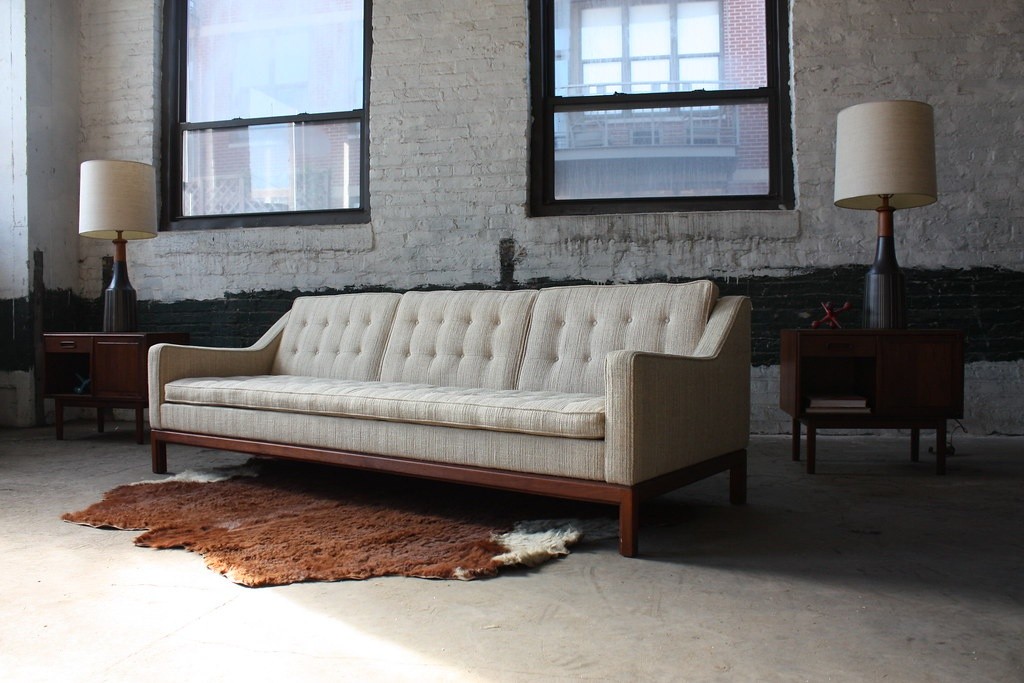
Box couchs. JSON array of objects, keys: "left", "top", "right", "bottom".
[{"left": 147, "top": 280, "right": 751, "bottom": 559}]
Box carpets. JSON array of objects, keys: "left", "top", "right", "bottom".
[{"left": 60, "top": 451, "right": 619, "bottom": 588}]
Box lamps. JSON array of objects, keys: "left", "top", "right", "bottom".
[
  {"left": 834, "top": 100, "right": 938, "bottom": 329},
  {"left": 77, "top": 159, "right": 158, "bottom": 332}
]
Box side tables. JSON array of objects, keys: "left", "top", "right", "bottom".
[
  {"left": 779, "top": 327, "right": 966, "bottom": 476},
  {"left": 41, "top": 334, "right": 190, "bottom": 445}
]
